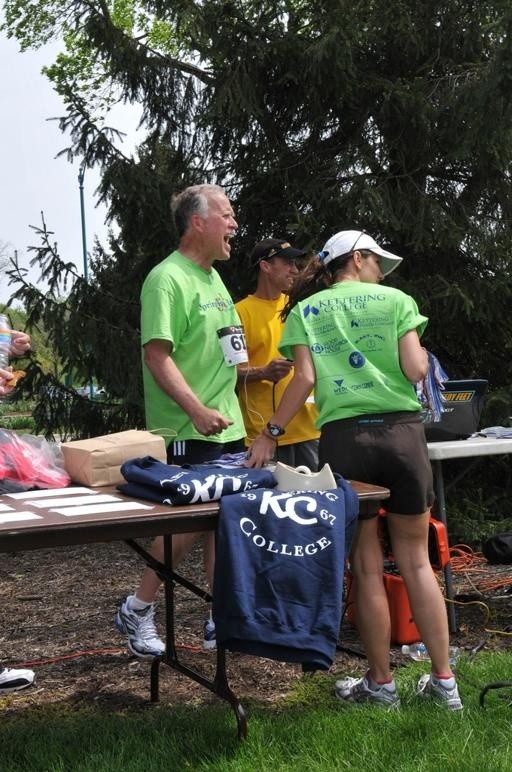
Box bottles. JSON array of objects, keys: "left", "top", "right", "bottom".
[
  {"left": 400, "top": 639, "right": 462, "bottom": 665},
  {"left": 0, "top": 311, "right": 13, "bottom": 375}
]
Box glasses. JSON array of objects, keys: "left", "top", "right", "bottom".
[{"left": 347, "top": 228, "right": 370, "bottom": 256}]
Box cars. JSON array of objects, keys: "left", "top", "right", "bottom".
[{"left": 39, "top": 385, "right": 107, "bottom": 427}]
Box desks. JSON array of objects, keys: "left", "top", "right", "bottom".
[
  {"left": 427, "top": 439, "right": 512, "bottom": 633},
  {"left": 0, "top": 480, "right": 390, "bottom": 744}
]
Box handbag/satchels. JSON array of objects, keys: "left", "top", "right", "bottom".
[{"left": 58, "top": 425, "right": 179, "bottom": 492}]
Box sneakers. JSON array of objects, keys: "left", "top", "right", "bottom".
[
  {"left": 0, "top": 665, "right": 37, "bottom": 693},
  {"left": 202, "top": 617, "right": 221, "bottom": 650},
  {"left": 331, "top": 668, "right": 402, "bottom": 709},
  {"left": 112, "top": 595, "right": 166, "bottom": 659},
  {"left": 415, "top": 672, "right": 463, "bottom": 714}
]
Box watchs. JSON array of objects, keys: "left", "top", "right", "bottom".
[{"left": 266, "top": 422, "right": 286, "bottom": 437}]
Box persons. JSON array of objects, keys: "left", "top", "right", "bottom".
[
  {"left": 234, "top": 238, "right": 321, "bottom": 471},
  {"left": 116, "top": 184, "right": 249, "bottom": 658},
  {"left": 241, "top": 230, "right": 466, "bottom": 711},
  {"left": 0, "top": 328, "right": 37, "bottom": 691}
]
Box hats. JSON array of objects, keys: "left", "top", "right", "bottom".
[
  {"left": 248, "top": 236, "right": 310, "bottom": 269},
  {"left": 319, "top": 229, "right": 403, "bottom": 280}
]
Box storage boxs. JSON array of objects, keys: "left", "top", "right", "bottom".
[{"left": 347, "top": 511, "right": 450, "bottom": 645}]
